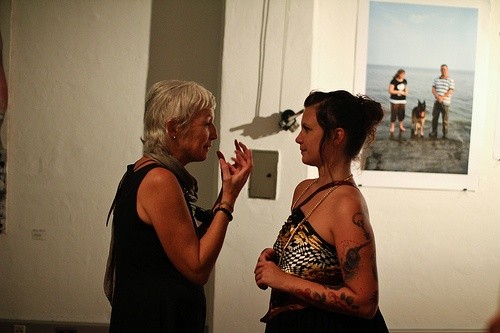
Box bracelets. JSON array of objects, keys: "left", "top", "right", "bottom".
[
  {"left": 219, "top": 201, "right": 234, "bottom": 213},
  {"left": 214, "top": 207, "right": 233, "bottom": 222}
]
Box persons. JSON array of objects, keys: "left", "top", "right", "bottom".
[
  {"left": 388, "top": 69, "right": 408, "bottom": 133},
  {"left": 103, "top": 81, "right": 253, "bottom": 333},
  {"left": 430, "top": 65, "right": 454, "bottom": 139},
  {"left": 0, "top": 31, "right": 8, "bottom": 235},
  {"left": 254, "top": 90, "right": 389, "bottom": 333}
]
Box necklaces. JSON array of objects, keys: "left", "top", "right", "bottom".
[{"left": 278, "top": 174, "right": 353, "bottom": 266}]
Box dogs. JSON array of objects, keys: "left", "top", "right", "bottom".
[{"left": 410, "top": 98, "right": 426, "bottom": 137}]
